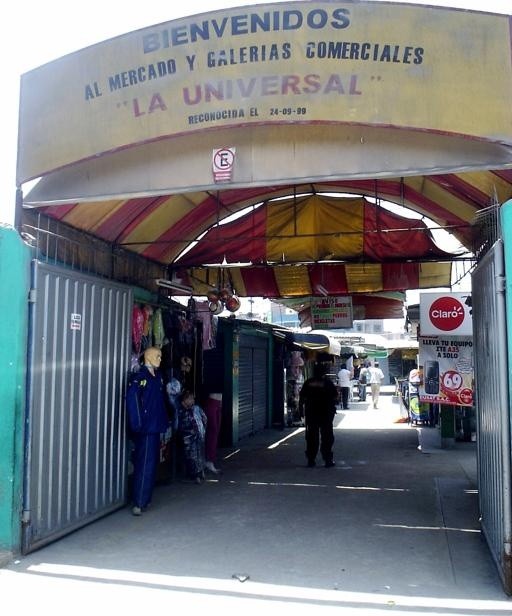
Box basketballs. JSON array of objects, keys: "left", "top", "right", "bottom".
[
  {"left": 207, "top": 289, "right": 219, "bottom": 302},
  {"left": 210, "top": 300, "right": 224, "bottom": 314},
  {"left": 225, "top": 297, "right": 240, "bottom": 312},
  {"left": 219, "top": 289, "right": 232, "bottom": 302}
]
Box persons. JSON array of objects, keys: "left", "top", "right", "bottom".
[
  {"left": 125, "top": 345, "right": 171, "bottom": 520},
  {"left": 357, "top": 361, "right": 367, "bottom": 402},
  {"left": 337, "top": 363, "right": 352, "bottom": 411},
  {"left": 298, "top": 366, "right": 343, "bottom": 470},
  {"left": 179, "top": 390, "right": 208, "bottom": 487},
  {"left": 368, "top": 361, "right": 386, "bottom": 410}
]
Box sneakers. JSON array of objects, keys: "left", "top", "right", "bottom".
[
  {"left": 344, "top": 398, "right": 379, "bottom": 409},
  {"left": 308, "top": 460, "right": 336, "bottom": 468},
  {"left": 204, "top": 461, "right": 223, "bottom": 475}
]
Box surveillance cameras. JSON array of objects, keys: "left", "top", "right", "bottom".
[{"left": 317, "top": 284, "right": 328, "bottom": 297}]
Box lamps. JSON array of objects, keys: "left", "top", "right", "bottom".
[{"left": 155, "top": 277, "right": 194, "bottom": 295}]
